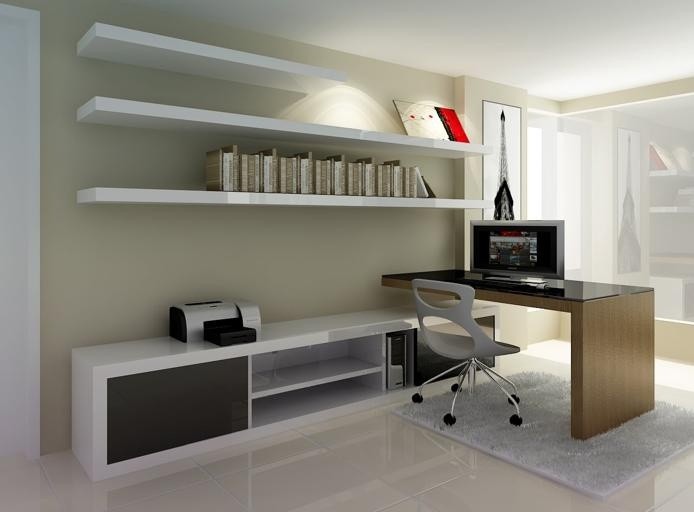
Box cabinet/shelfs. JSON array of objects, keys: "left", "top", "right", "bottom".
[
  {"left": 72, "top": 300, "right": 500, "bottom": 484},
  {"left": 648, "top": 169, "right": 694, "bottom": 214},
  {"left": 75, "top": 23, "right": 491, "bottom": 209},
  {"left": 650, "top": 276, "right": 694, "bottom": 321}
]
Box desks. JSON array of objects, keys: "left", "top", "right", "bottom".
[{"left": 382, "top": 269, "right": 655, "bottom": 439}]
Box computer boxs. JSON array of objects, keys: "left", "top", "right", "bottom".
[{"left": 386, "top": 332, "right": 407, "bottom": 390}]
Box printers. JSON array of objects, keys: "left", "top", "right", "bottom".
[{"left": 169, "top": 301, "right": 262, "bottom": 346}]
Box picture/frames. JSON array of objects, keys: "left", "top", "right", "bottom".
[{"left": 482, "top": 100, "right": 523, "bottom": 221}]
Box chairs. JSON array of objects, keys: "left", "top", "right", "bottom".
[{"left": 413, "top": 278, "right": 523, "bottom": 428}]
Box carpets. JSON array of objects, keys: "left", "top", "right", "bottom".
[{"left": 394, "top": 370, "right": 694, "bottom": 498}]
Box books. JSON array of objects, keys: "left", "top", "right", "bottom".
[{"left": 205, "top": 144, "right": 437, "bottom": 198}]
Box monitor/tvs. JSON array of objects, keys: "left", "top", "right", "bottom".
[{"left": 470, "top": 220, "right": 564, "bottom": 283}]
